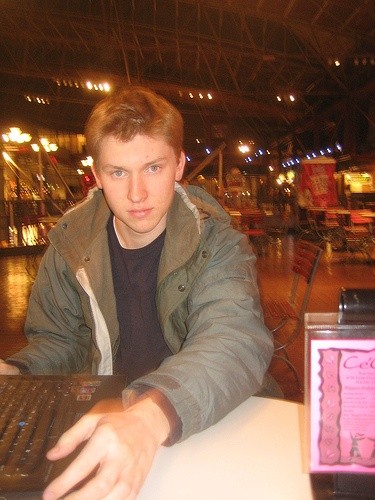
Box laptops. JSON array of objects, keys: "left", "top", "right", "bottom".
[{"left": 0, "top": 372, "right": 128, "bottom": 500}]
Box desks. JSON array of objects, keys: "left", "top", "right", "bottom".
[{"left": 138, "top": 396, "right": 311, "bottom": 500}]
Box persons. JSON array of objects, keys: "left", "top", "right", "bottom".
[{"left": 0, "top": 87, "right": 284, "bottom": 500}]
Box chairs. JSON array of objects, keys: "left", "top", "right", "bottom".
[{"left": 260, "top": 240, "right": 323, "bottom": 388}]
